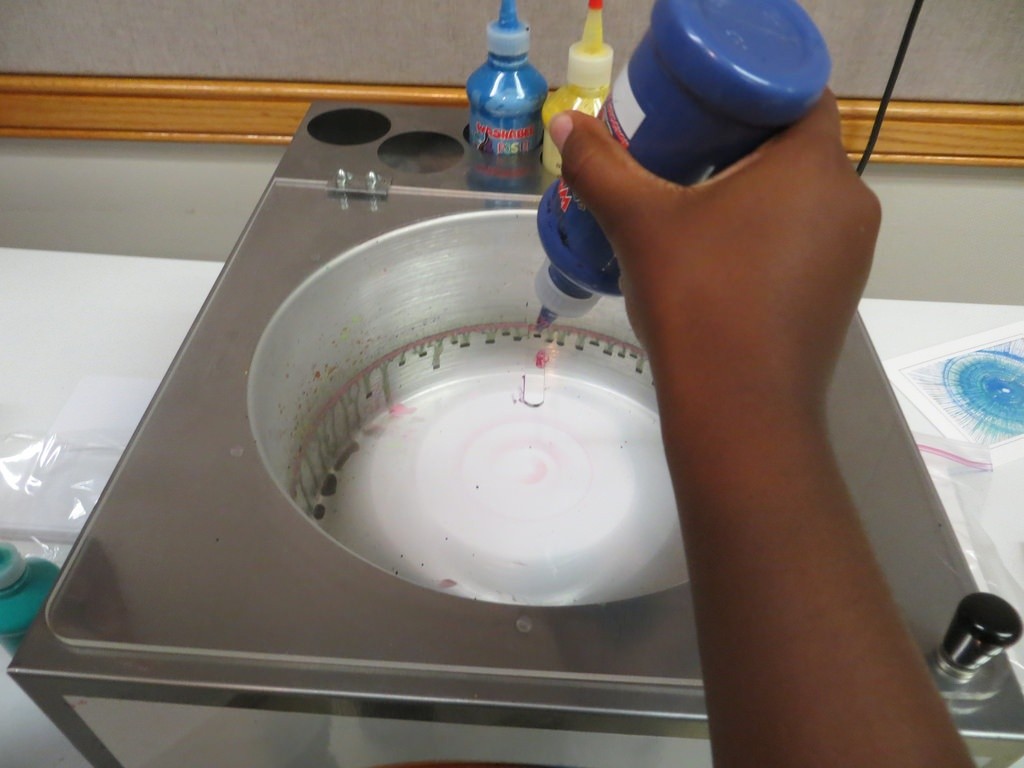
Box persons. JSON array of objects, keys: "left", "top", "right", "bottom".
[{"left": 548, "top": 84, "right": 980, "bottom": 768}]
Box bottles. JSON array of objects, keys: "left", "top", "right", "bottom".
[
  {"left": 530, "top": 0, "right": 830, "bottom": 329},
  {"left": 464, "top": 0, "right": 548, "bottom": 194},
  {"left": 1, "top": 542, "right": 61, "bottom": 654},
  {"left": 540, "top": 0, "right": 615, "bottom": 195}
]
